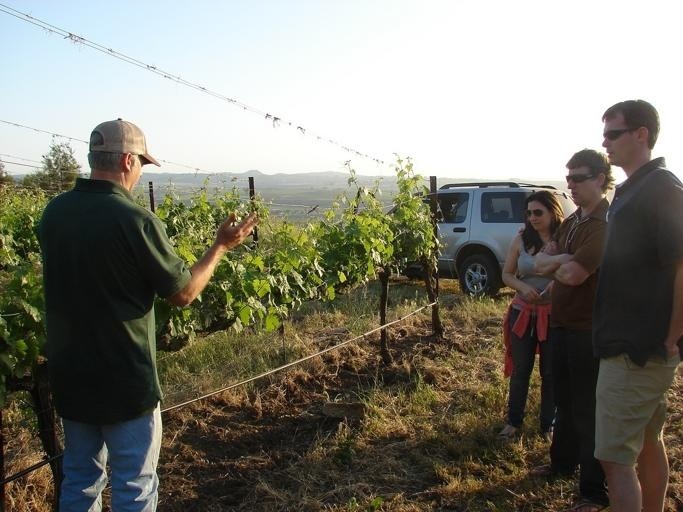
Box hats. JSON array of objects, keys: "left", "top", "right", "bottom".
[{"left": 89, "top": 117, "right": 161, "bottom": 168}]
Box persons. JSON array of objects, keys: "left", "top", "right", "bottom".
[
  {"left": 522, "top": 146, "right": 619, "bottom": 510},
  {"left": 31, "top": 117, "right": 261, "bottom": 511},
  {"left": 497, "top": 190, "right": 565, "bottom": 444},
  {"left": 591, "top": 99, "right": 681, "bottom": 512}
]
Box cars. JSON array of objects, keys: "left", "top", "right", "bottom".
[{"left": 386, "top": 181, "right": 579, "bottom": 296}]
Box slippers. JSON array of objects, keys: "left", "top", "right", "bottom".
[
  {"left": 498, "top": 424, "right": 516, "bottom": 440},
  {"left": 530, "top": 463, "right": 558, "bottom": 476},
  {"left": 570, "top": 498, "right": 604, "bottom": 512}
]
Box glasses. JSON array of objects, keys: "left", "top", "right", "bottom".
[
  {"left": 525, "top": 209, "right": 543, "bottom": 219},
  {"left": 602, "top": 129, "right": 638, "bottom": 140},
  {"left": 566, "top": 174, "right": 599, "bottom": 184}
]
